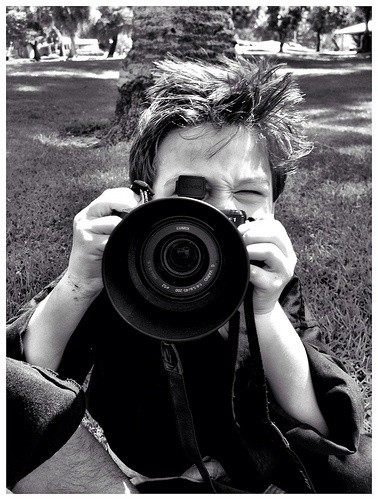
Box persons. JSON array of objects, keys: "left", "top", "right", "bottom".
[{"left": 5, "top": 48, "right": 371, "bottom": 495}]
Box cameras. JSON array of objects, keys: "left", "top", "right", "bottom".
[{"left": 97, "top": 170, "right": 252, "bottom": 342}]
